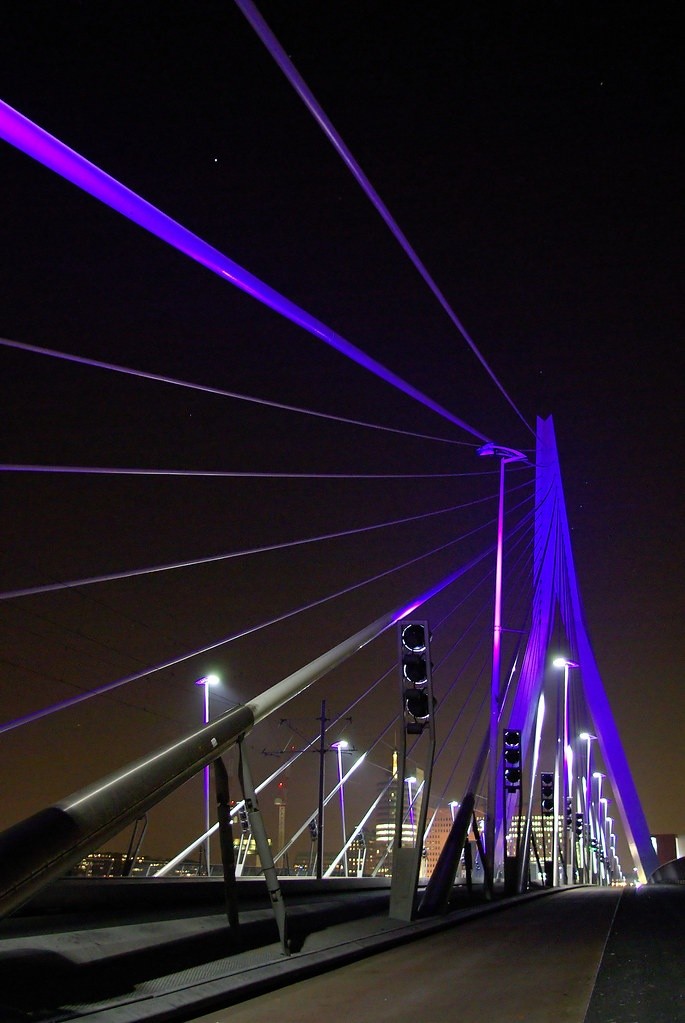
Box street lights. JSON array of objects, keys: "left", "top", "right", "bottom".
[
  {"left": 195, "top": 674, "right": 219, "bottom": 876},
  {"left": 403, "top": 777, "right": 418, "bottom": 848},
  {"left": 448, "top": 800, "right": 458, "bottom": 823},
  {"left": 579, "top": 733, "right": 593, "bottom": 884},
  {"left": 330, "top": 741, "right": 349, "bottom": 876},
  {"left": 593, "top": 772, "right": 602, "bottom": 829},
  {"left": 600, "top": 797, "right": 607, "bottom": 847},
  {"left": 605, "top": 816, "right": 612, "bottom": 868}
]
